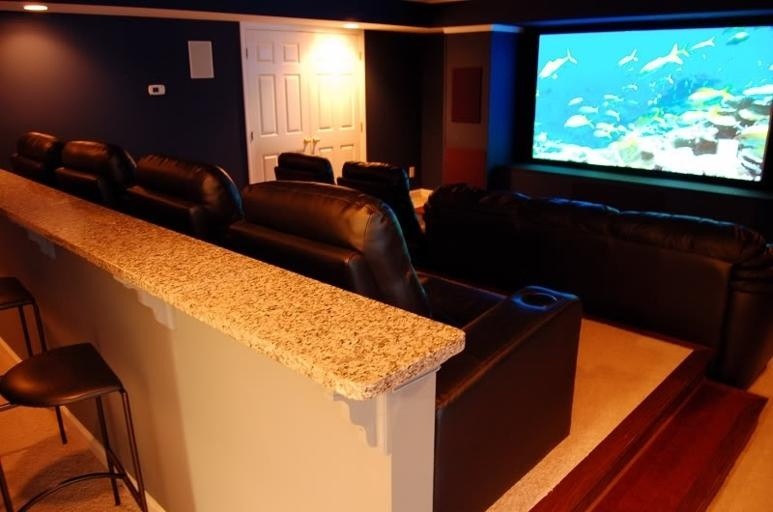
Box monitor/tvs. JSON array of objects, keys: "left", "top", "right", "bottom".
[{"left": 527, "top": 22, "right": 773, "bottom": 188}]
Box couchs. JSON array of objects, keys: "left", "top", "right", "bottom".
[
  {"left": 336, "top": 160, "right": 422, "bottom": 266},
  {"left": 124, "top": 153, "right": 243, "bottom": 243},
  {"left": 54, "top": 141, "right": 129, "bottom": 212},
  {"left": 275, "top": 154, "right": 336, "bottom": 184},
  {"left": 230, "top": 182, "right": 582, "bottom": 512},
  {"left": 3, "top": 132, "right": 67, "bottom": 182},
  {"left": 420, "top": 181, "right": 772, "bottom": 392}
]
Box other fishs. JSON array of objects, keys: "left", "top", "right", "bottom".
[
  {"left": 565, "top": 31, "right": 773, "bottom": 184},
  {"left": 541, "top": 48, "right": 575, "bottom": 79}
]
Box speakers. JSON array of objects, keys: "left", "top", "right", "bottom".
[{"left": 187, "top": 40, "right": 215, "bottom": 79}]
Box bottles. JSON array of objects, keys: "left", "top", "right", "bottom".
[{"left": 408, "top": 167, "right": 418, "bottom": 189}]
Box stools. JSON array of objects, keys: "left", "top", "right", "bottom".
[
  {"left": 1, "top": 278, "right": 68, "bottom": 444},
  {"left": 1, "top": 342, "right": 148, "bottom": 512}
]
